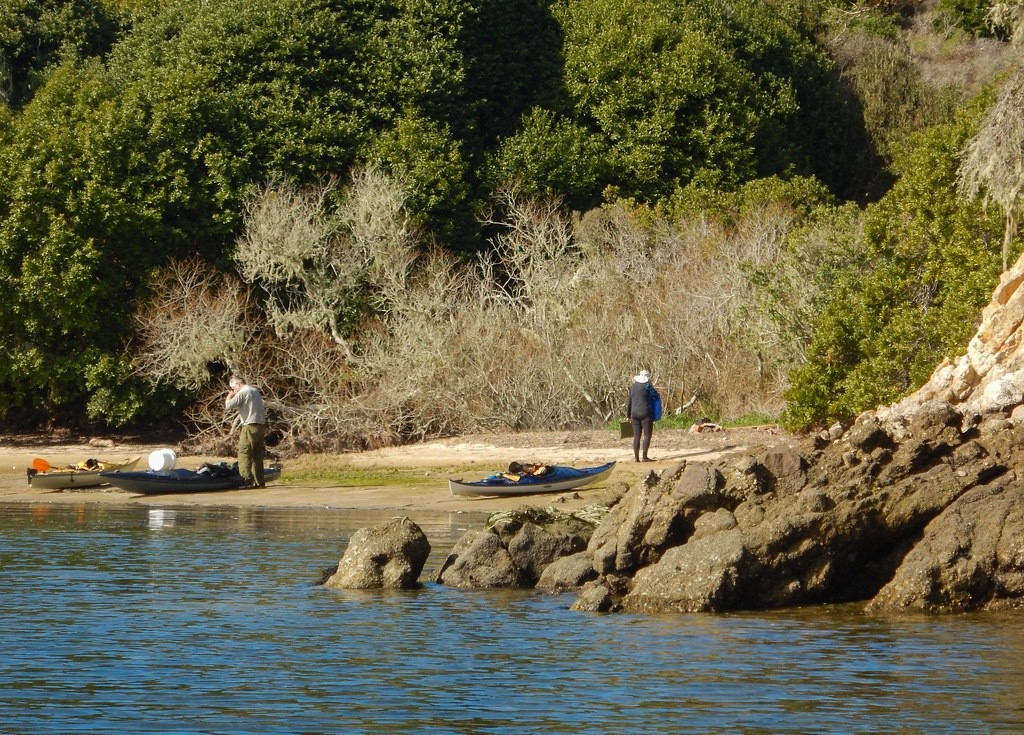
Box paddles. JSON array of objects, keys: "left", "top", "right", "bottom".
[{"left": 32, "top": 458, "right": 59, "bottom": 471}]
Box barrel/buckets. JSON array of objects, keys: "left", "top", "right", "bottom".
[{"left": 150, "top": 448, "right": 176, "bottom": 471}]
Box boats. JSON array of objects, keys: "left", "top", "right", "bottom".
[
  {"left": 96, "top": 463, "right": 281, "bottom": 493},
  {"left": 26, "top": 457, "right": 141, "bottom": 487},
  {"left": 447, "top": 461, "right": 619, "bottom": 496}
]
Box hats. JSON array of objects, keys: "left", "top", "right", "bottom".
[{"left": 633, "top": 370, "right": 651, "bottom": 383}]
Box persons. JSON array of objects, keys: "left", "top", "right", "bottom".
[
  {"left": 627, "top": 370, "right": 653, "bottom": 462},
  {"left": 225, "top": 376, "right": 265, "bottom": 488}
]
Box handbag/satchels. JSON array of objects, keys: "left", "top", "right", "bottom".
[{"left": 646, "top": 384, "right": 661, "bottom": 421}]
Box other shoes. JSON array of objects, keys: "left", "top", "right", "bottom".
[
  {"left": 635, "top": 459, "right": 657, "bottom": 462},
  {"left": 235, "top": 478, "right": 266, "bottom": 489}
]
